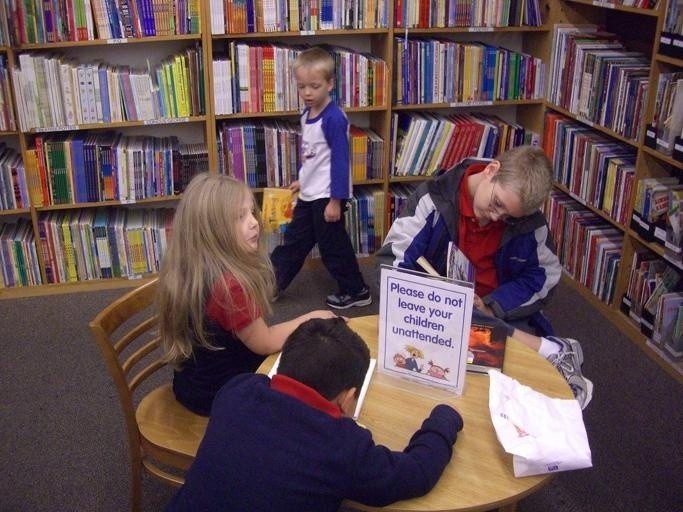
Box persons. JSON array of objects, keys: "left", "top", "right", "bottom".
[{"left": 164, "top": 316, "right": 463, "bottom": 512}]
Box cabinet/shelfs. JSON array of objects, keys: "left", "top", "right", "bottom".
[
  {"left": 1, "top": 0, "right": 212, "bottom": 298},
  {"left": 214, "top": 2, "right": 556, "bottom": 268},
  {"left": 541, "top": 0, "right": 683, "bottom": 390}
]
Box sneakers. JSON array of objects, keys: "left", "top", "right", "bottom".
[
  {"left": 326, "top": 288, "right": 372, "bottom": 309},
  {"left": 546, "top": 336, "right": 593, "bottom": 411}
]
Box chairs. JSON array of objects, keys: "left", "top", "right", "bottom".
[{"left": 88, "top": 274, "right": 212, "bottom": 511}]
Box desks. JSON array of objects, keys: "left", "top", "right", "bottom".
[{"left": 252, "top": 312, "right": 573, "bottom": 512}]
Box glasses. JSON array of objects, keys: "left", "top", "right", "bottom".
[{"left": 489, "top": 178, "right": 514, "bottom": 225}]
